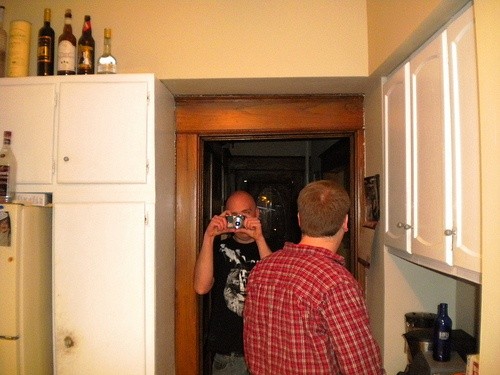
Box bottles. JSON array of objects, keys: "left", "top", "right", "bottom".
[
  {"left": 36, "top": 8, "right": 55, "bottom": 76},
  {"left": 0, "top": 131, "right": 17, "bottom": 204},
  {"left": 56, "top": 8, "right": 77, "bottom": 75},
  {"left": 77, "top": 15, "right": 95, "bottom": 75},
  {"left": 0, "top": 5, "right": 7, "bottom": 77},
  {"left": 433, "top": 303, "right": 452, "bottom": 362},
  {"left": 97, "top": 28, "right": 117, "bottom": 74}
]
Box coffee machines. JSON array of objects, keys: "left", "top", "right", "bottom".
[{"left": 405, "top": 312, "right": 438, "bottom": 362}]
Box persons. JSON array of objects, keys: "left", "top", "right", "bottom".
[
  {"left": 194, "top": 189, "right": 273, "bottom": 375},
  {"left": 0, "top": 211, "right": 11, "bottom": 247},
  {"left": 243, "top": 180, "right": 386, "bottom": 375}
]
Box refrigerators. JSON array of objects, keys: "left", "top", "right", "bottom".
[{"left": 0, "top": 201, "right": 54, "bottom": 375}]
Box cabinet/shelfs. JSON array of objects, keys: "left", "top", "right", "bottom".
[
  {"left": 381, "top": 28, "right": 451, "bottom": 262},
  {"left": 0, "top": 73, "right": 178, "bottom": 375},
  {"left": 448, "top": 6, "right": 482, "bottom": 284}
]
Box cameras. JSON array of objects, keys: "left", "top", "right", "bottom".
[{"left": 226, "top": 215, "right": 244, "bottom": 229}]
